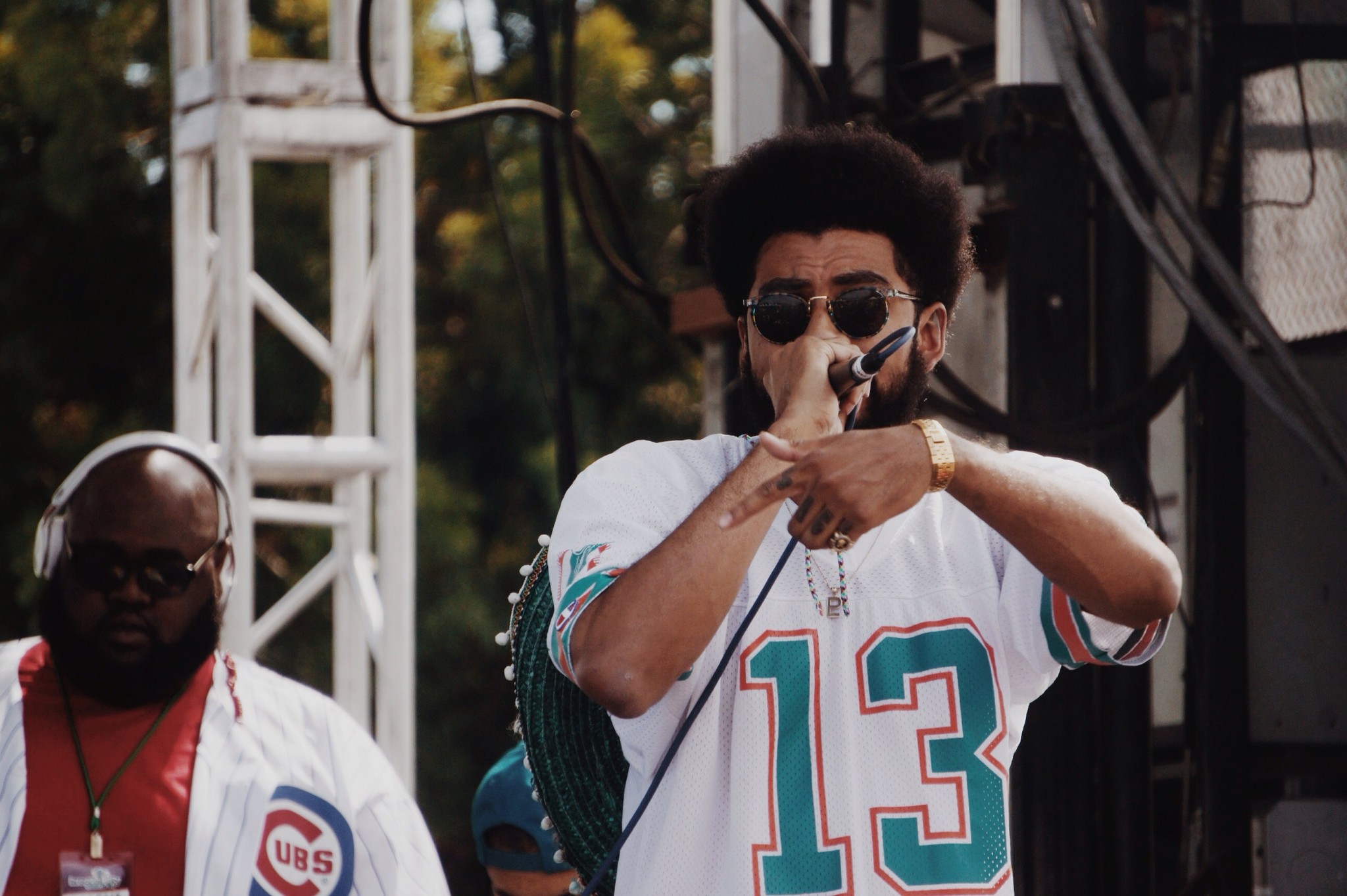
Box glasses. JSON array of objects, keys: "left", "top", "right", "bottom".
[
  {"left": 59, "top": 539, "right": 208, "bottom": 599},
  {"left": 740, "top": 286, "right": 922, "bottom": 346}
]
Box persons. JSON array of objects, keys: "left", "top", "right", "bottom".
[
  {"left": 0, "top": 430, "right": 451, "bottom": 896},
  {"left": 547, "top": 121, "right": 1182, "bottom": 896},
  {"left": 472, "top": 741, "right": 596, "bottom": 896}
]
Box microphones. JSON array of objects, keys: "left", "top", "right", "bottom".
[{"left": 829, "top": 354, "right": 885, "bottom": 398}]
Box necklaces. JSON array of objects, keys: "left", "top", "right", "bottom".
[{"left": 783, "top": 500, "right": 885, "bottom": 617}]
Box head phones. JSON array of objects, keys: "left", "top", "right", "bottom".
[{"left": 33, "top": 429, "right": 236, "bottom": 606}]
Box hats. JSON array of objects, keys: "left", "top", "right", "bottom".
[{"left": 494, "top": 532, "right": 631, "bottom": 893}]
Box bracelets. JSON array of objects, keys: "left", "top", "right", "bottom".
[{"left": 912, "top": 419, "right": 955, "bottom": 493}]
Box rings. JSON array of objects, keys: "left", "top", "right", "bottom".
[{"left": 828, "top": 531, "right": 855, "bottom": 552}]
